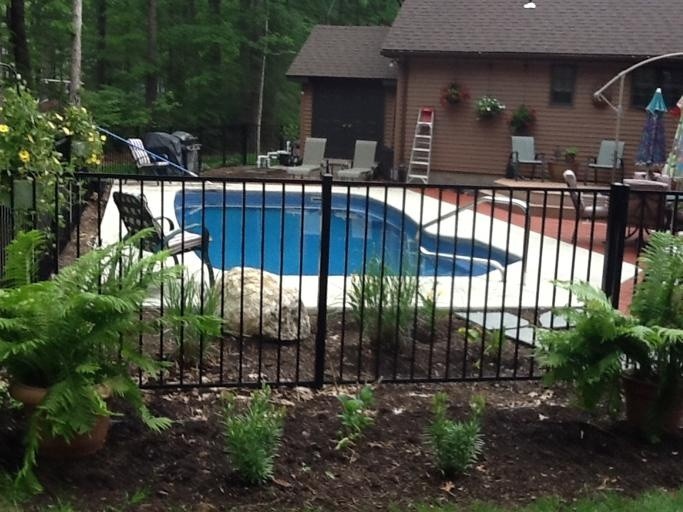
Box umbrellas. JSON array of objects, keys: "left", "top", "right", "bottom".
[
  {"left": 634, "top": 88, "right": 668, "bottom": 179},
  {"left": 662, "top": 94, "right": 683, "bottom": 255}
]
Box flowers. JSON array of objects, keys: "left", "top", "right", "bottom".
[{"left": 438, "top": 80, "right": 535, "bottom": 132}]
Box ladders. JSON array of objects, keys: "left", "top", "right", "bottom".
[{"left": 404, "top": 105, "right": 435, "bottom": 185}]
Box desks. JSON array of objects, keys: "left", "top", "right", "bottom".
[{"left": 618, "top": 179, "right": 670, "bottom": 248}]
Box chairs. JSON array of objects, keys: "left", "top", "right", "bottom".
[
  {"left": 507, "top": 134, "right": 625, "bottom": 184},
  {"left": 107, "top": 138, "right": 214, "bottom": 291},
  {"left": 286, "top": 137, "right": 377, "bottom": 182},
  {"left": 563, "top": 168, "right": 610, "bottom": 242}
]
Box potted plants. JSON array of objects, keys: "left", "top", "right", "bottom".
[
  {"left": 0, "top": 224, "right": 226, "bottom": 507},
  {"left": 535, "top": 229, "right": 683, "bottom": 442}
]
